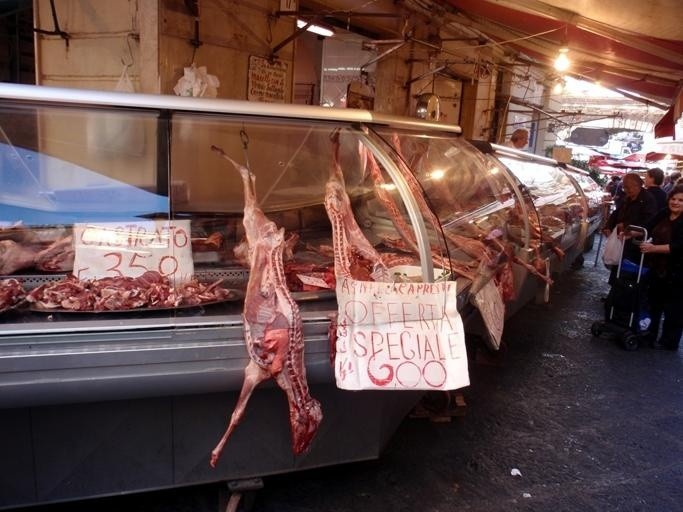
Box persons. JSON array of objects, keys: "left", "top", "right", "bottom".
[
  {"left": 602, "top": 168, "right": 682, "bottom": 354},
  {"left": 500, "top": 127, "right": 530, "bottom": 151}
]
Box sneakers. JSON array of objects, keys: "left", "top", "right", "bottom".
[{"left": 639, "top": 336, "right": 679, "bottom": 351}]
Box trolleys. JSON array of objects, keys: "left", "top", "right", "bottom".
[{"left": 591, "top": 224, "right": 652, "bottom": 352}]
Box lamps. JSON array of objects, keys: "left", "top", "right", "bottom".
[{"left": 553, "top": 11, "right": 573, "bottom": 74}]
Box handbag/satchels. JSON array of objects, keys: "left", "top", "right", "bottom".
[{"left": 600, "top": 225, "right": 625, "bottom": 266}]
